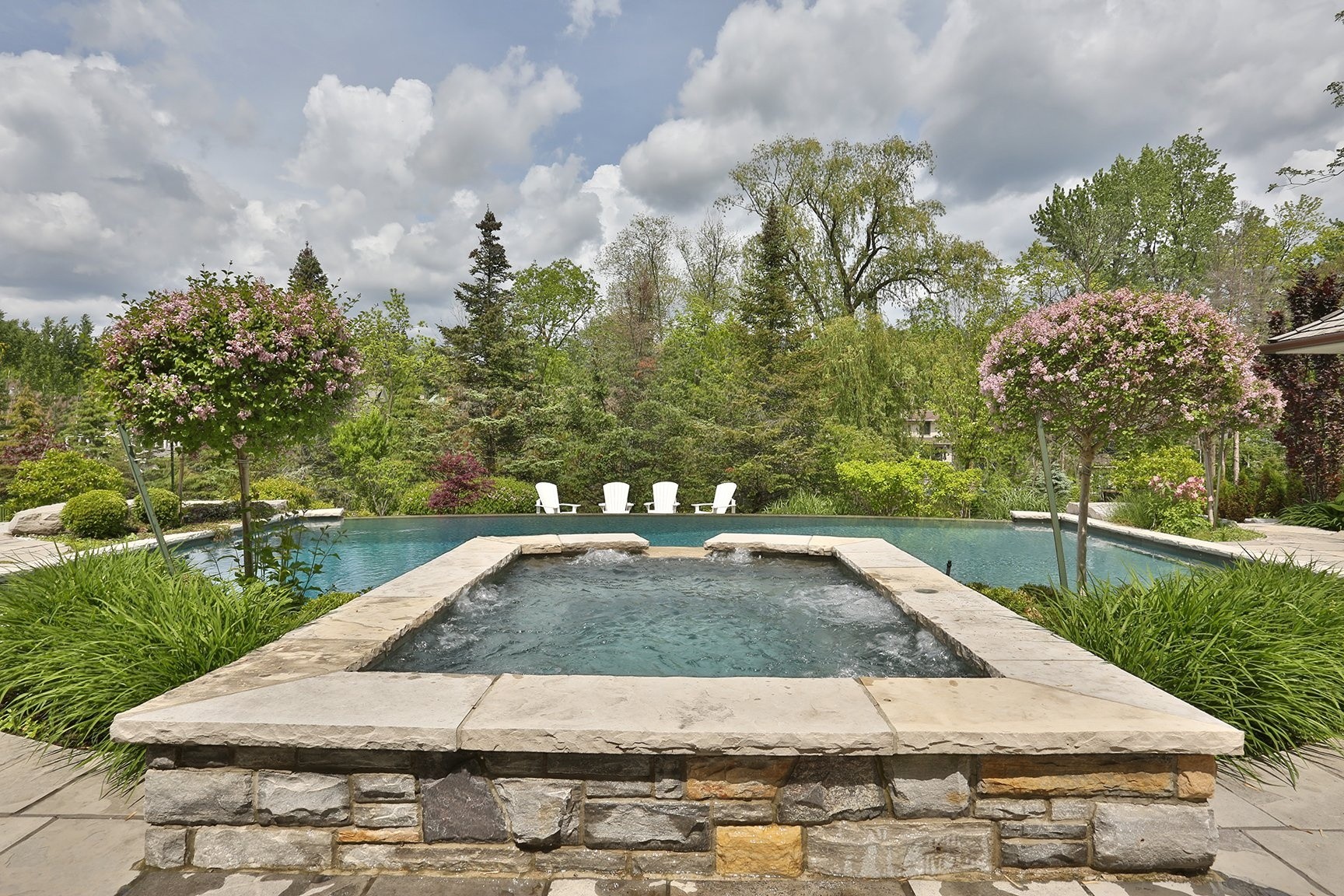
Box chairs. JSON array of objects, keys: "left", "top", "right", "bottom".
[
  {"left": 643, "top": 481, "right": 681, "bottom": 513},
  {"left": 535, "top": 482, "right": 581, "bottom": 513},
  {"left": 691, "top": 482, "right": 737, "bottom": 513},
  {"left": 598, "top": 481, "right": 635, "bottom": 513}
]
